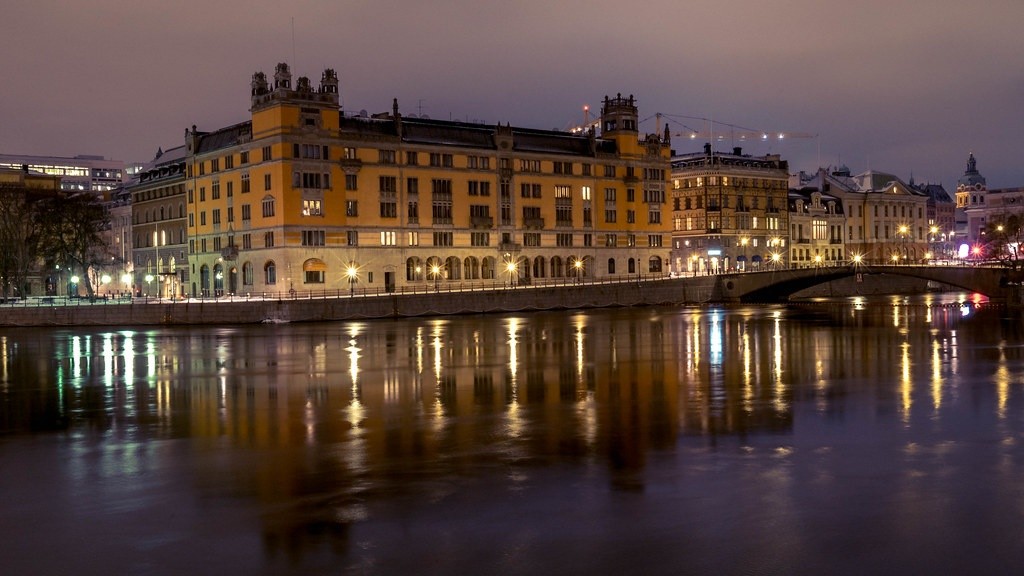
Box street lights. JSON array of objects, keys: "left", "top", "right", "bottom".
[
  {"left": 347, "top": 267, "right": 357, "bottom": 297},
  {"left": 145, "top": 275, "right": 154, "bottom": 297},
  {"left": 693, "top": 255, "right": 699, "bottom": 276},
  {"left": 101, "top": 275, "right": 110, "bottom": 300},
  {"left": 509, "top": 263, "right": 514, "bottom": 288},
  {"left": 432, "top": 267, "right": 440, "bottom": 294},
  {"left": 932, "top": 225, "right": 938, "bottom": 261},
  {"left": 901, "top": 226, "right": 907, "bottom": 260},
  {"left": 413, "top": 266, "right": 422, "bottom": 294},
  {"left": 575, "top": 260, "right": 582, "bottom": 284}
]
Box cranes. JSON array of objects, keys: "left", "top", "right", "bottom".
[
  {"left": 638, "top": 112, "right": 814, "bottom": 140},
  {"left": 567, "top": 103, "right": 602, "bottom": 135}
]
